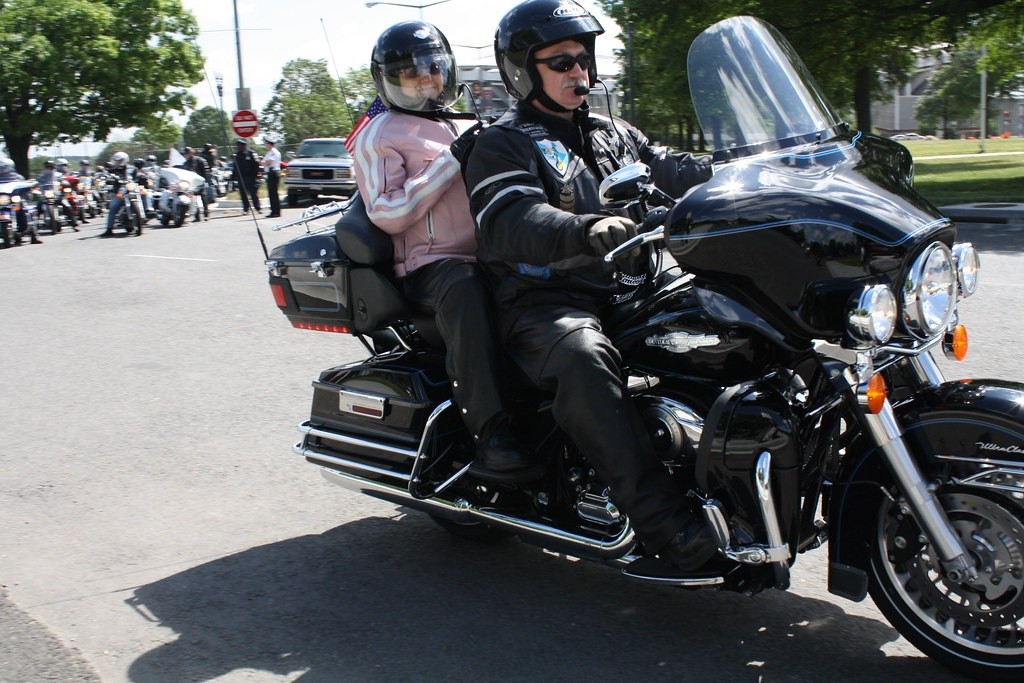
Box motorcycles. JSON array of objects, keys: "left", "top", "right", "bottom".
[
  {"left": 0, "top": 165, "right": 236, "bottom": 247},
  {"left": 266, "top": 15, "right": 1023, "bottom": 683}
]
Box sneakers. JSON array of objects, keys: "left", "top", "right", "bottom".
[
  {"left": 662, "top": 525, "right": 721, "bottom": 571},
  {"left": 477, "top": 419, "right": 529, "bottom": 473}
]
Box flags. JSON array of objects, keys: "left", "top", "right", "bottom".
[{"left": 343, "top": 95, "right": 389, "bottom": 160}]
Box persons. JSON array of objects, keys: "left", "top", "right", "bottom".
[
  {"left": 465, "top": 0, "right": 714, "bottom": 566},
  {"left": 943, "top": 120, "right": 960, "bottom": 139},
  {"left": 260, "top": 136, "right": 281, "bottom": 218},
  {"left": 1, "top": 136, "right": 264, "bottom": 244},
  {"left": 352, "top": 20, "right": 534, "bottom": 473}
]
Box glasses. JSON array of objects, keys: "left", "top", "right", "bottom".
[
  {"left": 398, "top": 66, "right": 443, "bottom": 81},
  {"left": 533, "top": 55, "right": 592, "bottom": 73}
]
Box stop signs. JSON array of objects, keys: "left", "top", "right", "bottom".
[{"left": 230, "top": 110, "right": 258, "bottom": 141}]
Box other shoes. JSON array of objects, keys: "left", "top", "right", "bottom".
[
  {"left": 266, "top": 213, "right": 281, "bottom": 218},
  {"left": 31, "top": 237, "right": 42, "bottom": 244},
  {"left": 193, "top": 218, "right": 201, "bottom": 222},
  {"left": 100, "top": 230, "right": 113, "bottom": 237}
]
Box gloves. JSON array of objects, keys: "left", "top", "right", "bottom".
[
  {"left": 589, "top": 216, "right": 641, "bottom": 262},
  {"left": 450, "top": 122, "right": 488, "bottom": 162}
]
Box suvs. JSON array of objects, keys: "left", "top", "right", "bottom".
[{"left": 286, "top": 137, "right": 357, "bottom": 204}]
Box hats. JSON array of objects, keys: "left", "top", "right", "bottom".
[
  {"left": 236, "top": 140, "right": 248, "bottom": 147},
  {"left": 263, "top": 136, "right": 278, "bottom": 145}
]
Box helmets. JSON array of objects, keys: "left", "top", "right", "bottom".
[
  {"left": 371, "top": 21, "right": 458, "bottom": 110},
  {"left": 146, "top": 155, "right": 157, "bottom": 164},
  {"left": 44, "top": 161, "right": 54, "bottom": 167},
  {"left": 0, "top": 158, "right": 16, "bottom": 173},
  {"left": 79, "top": 160, "right": 91, "bottom": 166},
  {"left": 57, "top": 158, "right": 69, "bottom": 166},
  {"left": 112, "top": 152, "right": 130, "bottom": 166},
  {"left": 493, "top": 1, "right": 607, "bottom": 103}
]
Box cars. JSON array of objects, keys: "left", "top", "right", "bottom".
[{"left": 890, "top": 134, "right": 938, "bottom": 140}]
